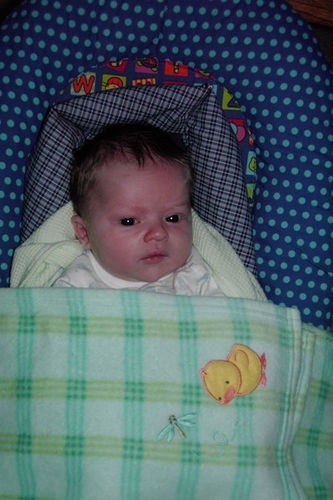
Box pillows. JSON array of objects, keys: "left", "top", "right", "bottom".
[{"left": 21, "top": 55, "right": 258, "bottom": 283}]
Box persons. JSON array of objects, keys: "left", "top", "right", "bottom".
[{"left": 52, "top": 121, "right": 230, "bottom": 298}]
